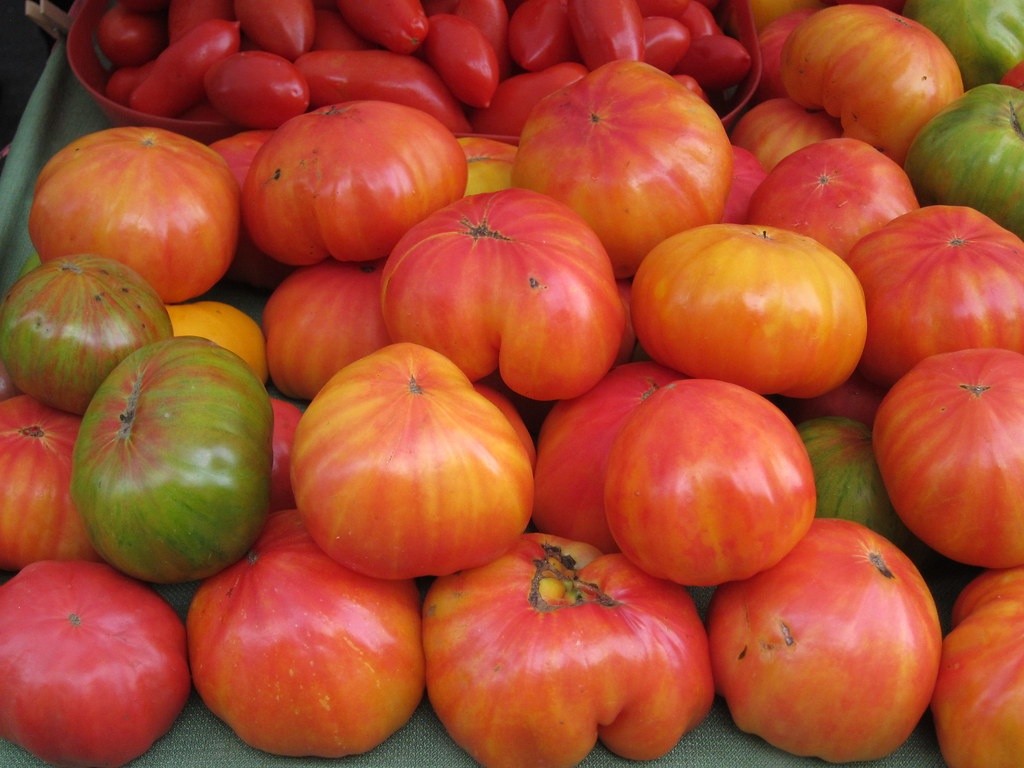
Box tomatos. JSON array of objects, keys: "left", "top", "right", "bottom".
[{"left": 0, "top": 0, "right": 1023, "bottom": 768}]
